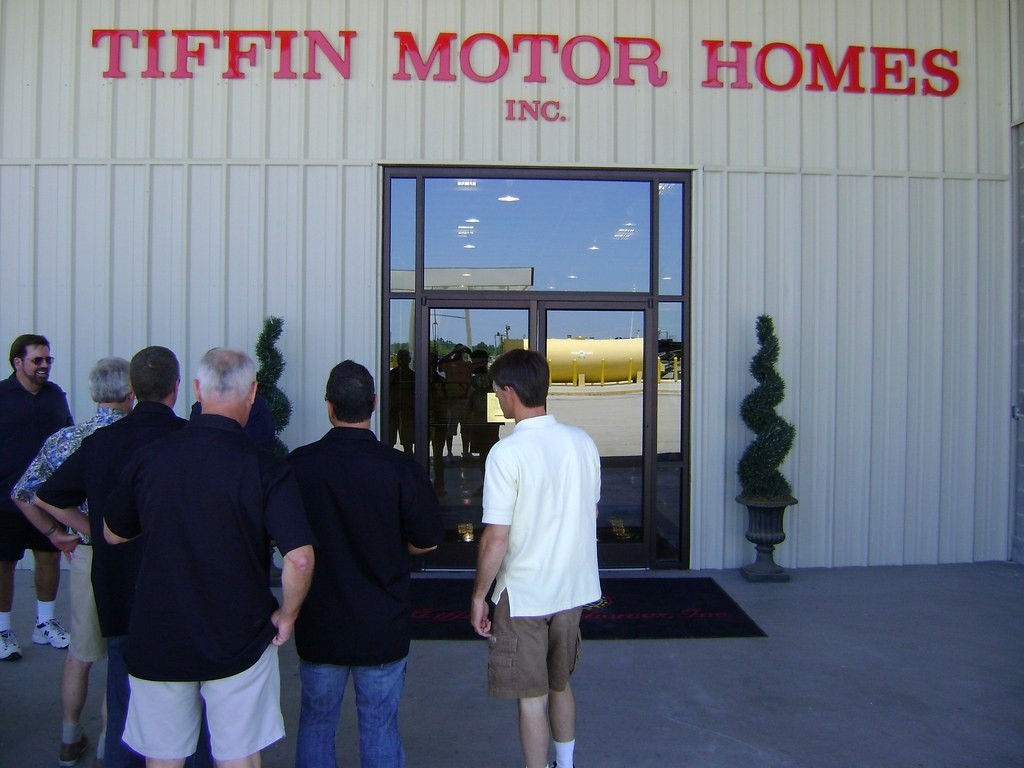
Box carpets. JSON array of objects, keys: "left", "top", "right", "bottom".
[
  {"left": 410, "top": 584, "right": 769, "bottom": 642},
  {"left": 440, "top": 503, "right": 679, "bottom": 545}
]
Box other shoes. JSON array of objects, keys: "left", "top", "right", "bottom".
[
  {"left": 59, "top": 735, "right": 88, "bottom": 767},
  {"left": 94, "top": 759, "right": 104, "bottom": 768}
]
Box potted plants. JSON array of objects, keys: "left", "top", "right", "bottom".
[{"left": 734, "top": 313, "right": 799, "bottom": 584}]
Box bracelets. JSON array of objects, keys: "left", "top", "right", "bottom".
[{"left": 43, "top": 522, "right": 57, "bottom": 537}]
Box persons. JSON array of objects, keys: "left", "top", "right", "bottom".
[
  {"left": 470, "top": 350, "right": 602, "bottom": 768},
  {"left": 0, "top": 334, "right": 445, "bottom": 767},
  {"left": 390, "top": 343, "right": 505, "bottom": 495}
]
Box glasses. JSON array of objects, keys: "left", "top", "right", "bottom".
[{"left": 23, "top": 357, "right": 54, "bottom": 365}]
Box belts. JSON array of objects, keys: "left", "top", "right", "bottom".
[{"left": 77, "top": 537, "right": 92, "bottom": 546}]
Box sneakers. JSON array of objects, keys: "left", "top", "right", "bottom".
[
  {"left": 0, "top": 630, "right": 23, "bottom": 661},
  {"left": 32, "top": 616, "right": 70, "bottom": 648}
]
